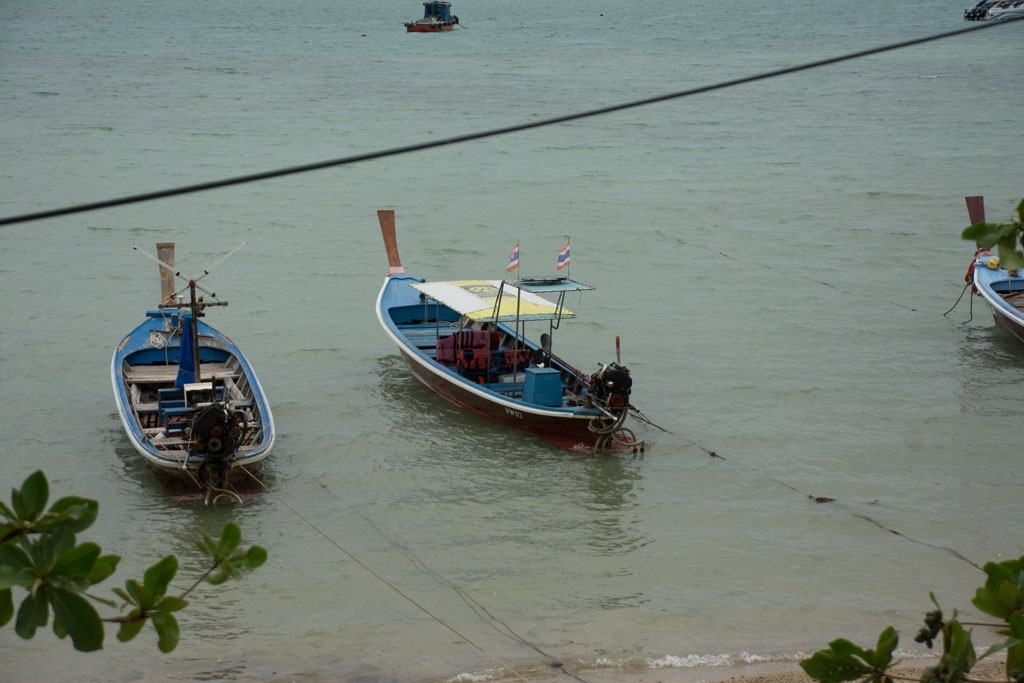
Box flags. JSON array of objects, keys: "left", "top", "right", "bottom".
[
  {"left": 506, "top": 243, "right": 519, "bottom": 273},
  {"left": 557, "top": 243, "right": 570, "bottom": 269}
]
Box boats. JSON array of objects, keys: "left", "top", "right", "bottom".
[
  {"left": 377, "top": 210, "right": 645, "bottom": 455},
  {"left": 965, "top": 196, "right": 1024, "bottom": 345},
  {"left": 402, "top": 0, "right": 459, "bottom": 32},
  {"left": 111, "top": 241, "right": 276, "bottom": 486}
]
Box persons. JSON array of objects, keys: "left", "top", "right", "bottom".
[
  {"left": 965, "top": 1, "right": 988, "bottom": 21},
  {"left": 480, "top": 322, "right": 500, "bottom": 350},
  {"left": 532, "top": 334, "right": 551, "bottom": 367}
]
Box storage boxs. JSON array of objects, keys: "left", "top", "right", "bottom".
[{"left": 498, "top": 371, "right": 526, "bottom": 381}]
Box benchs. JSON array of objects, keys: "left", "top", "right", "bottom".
[
  {"left": 144, "top": 421, "right": 259, "bottom": 437},
  {"left": 126, "top": 362, "right": 241, "bottom": 385},
  {"left": 133, "top": 397, "right": 253, "bottom": 412},
  {"left": 480, "top": 381, "right": 573, "bottom": 393}
]
